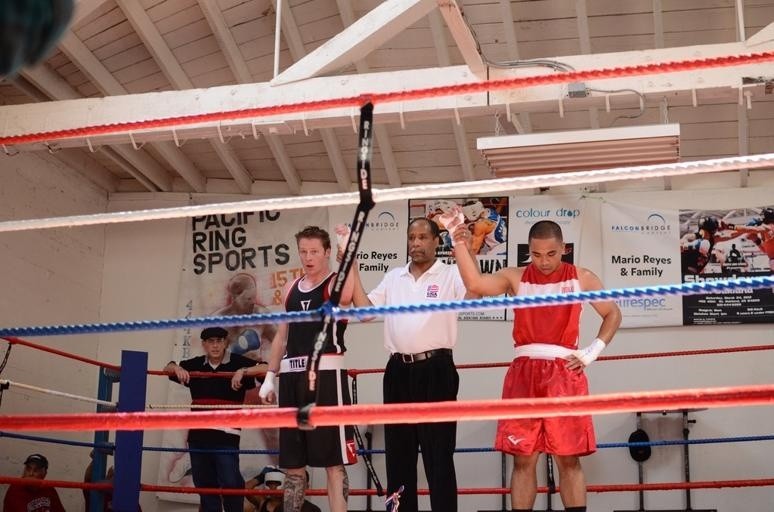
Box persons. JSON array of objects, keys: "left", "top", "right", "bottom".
[
  {"left": 83, "top": 448, "right": 142, "bottom": 512},
  {"left": 680, "top": 216, "right": 774, "bottom": 282},
  {"left": 164, "top": 327, "right": 267, "bottom": 512},
  {"left": 439, "top": 204, "right": 622, "bottom": 512},
  {"left": 258, "top": 223, "right": 354, "bottom": 512},
  {"left": 273, "top": 470, "right": 321, "bottom": 512},
  {"left": 244, "top": 465, "right": 286, "bottom": 512},
  {"left": 425, "top": 200, "right": 507, "bottom": 257},
  {"left": 3, "top": 454, "right": 66, "bottom": 512},
  {"left": 336, "top": 217, "right": 481, "bottom": 512},
  {"left": 168, "top": 273, "right": 279, "bottom": 483}
]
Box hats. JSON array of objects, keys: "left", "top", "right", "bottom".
[
  {"left": 200, "top": 327, "right": 228, "bottom": 340},
  {"left": 24, "top": 453, "right": 49, "bottom": 469}
]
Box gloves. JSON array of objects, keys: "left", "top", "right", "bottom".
[
  {"left": 746, "top": 232, "right": 761, "bottom": 246},
  {"left": 717, "top": 220, "right": 736, "bottom": 231},
  {"left": 230, "top": 328, "right": 262, "bottom": 356},
  {"left": 744, "top": 217, "right": 763, "bottom": 227},
  {"left": 253, "top": 465, "right": 277, "bottom": 484},
  {"left": 683, "top": 238, "right": 710, "bottom": 255}
]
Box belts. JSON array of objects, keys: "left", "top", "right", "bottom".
[{"left": 390, "top": 348, "right": 452, "bottom": 363}]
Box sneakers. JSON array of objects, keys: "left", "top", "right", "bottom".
[{"left": 169, "top": 452, "right": 191, "bottom": 483}]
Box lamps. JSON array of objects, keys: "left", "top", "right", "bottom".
[{"left": 466, "top": 96, "right": 683, "bottom": 177}]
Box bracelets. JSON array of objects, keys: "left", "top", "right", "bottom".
[{"left": 243, "top": 367, "right": 247, "bottom": 378}]
